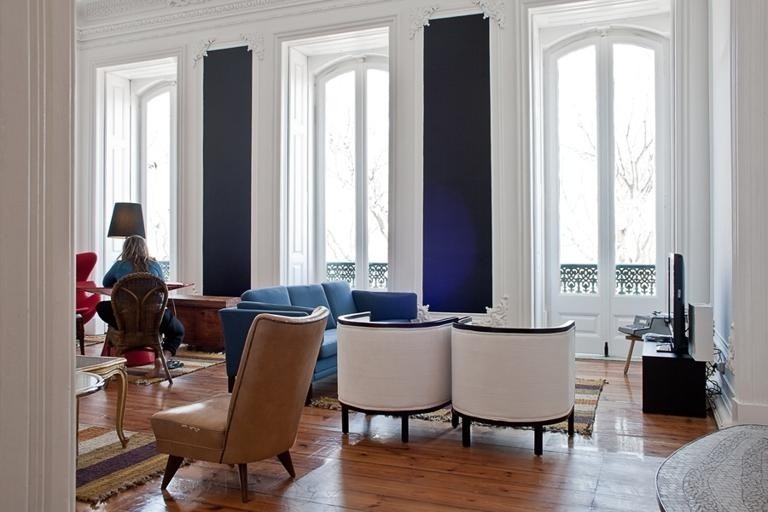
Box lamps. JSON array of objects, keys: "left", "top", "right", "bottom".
[{"left": 105, "top": 202, "right": 149, "bottom": 243}]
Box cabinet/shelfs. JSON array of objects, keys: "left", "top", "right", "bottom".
[{"left": 640, "top": 334, "right": 708, "bottom": 419}]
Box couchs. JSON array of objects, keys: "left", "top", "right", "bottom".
[{"left": 217, "top": 280, "right": 419, "bottom": 403}]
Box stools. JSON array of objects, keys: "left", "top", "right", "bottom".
[{"left": 166, "top": 294, "right": 242, "bottom": 352}]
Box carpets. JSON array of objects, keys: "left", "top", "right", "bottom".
[
  {"left": 77, "top": 331, "right": 227, "bottom": 385},
  {"left": 309, "top": 377, "right": 612, "bottom": 441},
  {"left": 75, "top": 420, "right": 196, "bottom": 510}
]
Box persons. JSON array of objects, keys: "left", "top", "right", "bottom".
[{"left": 95, "top": 234, "right": 187, "bottom": 371}]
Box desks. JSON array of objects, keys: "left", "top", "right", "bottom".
[
  {"left": 652, "top": 419, "right": 767, "bottom": 511},
  {"left": 74, "top": 353, "right": 136, "bottom": 456},
  {"left": 76, "top": 280, "right": 195, "bottom": 297},
  {"left": 624, "top": 335, "right": 675, "bottom": 375}
]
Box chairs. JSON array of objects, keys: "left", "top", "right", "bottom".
[
  {"left": 76, "top": 250, "right": 103, "bottom": 355},
  {"left": 105, "top": 271, "right": 175, "bottom": 390}
]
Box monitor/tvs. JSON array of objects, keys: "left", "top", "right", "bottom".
[{"left": 667, "top": 253, "right": 686, "bottom": 353}]
